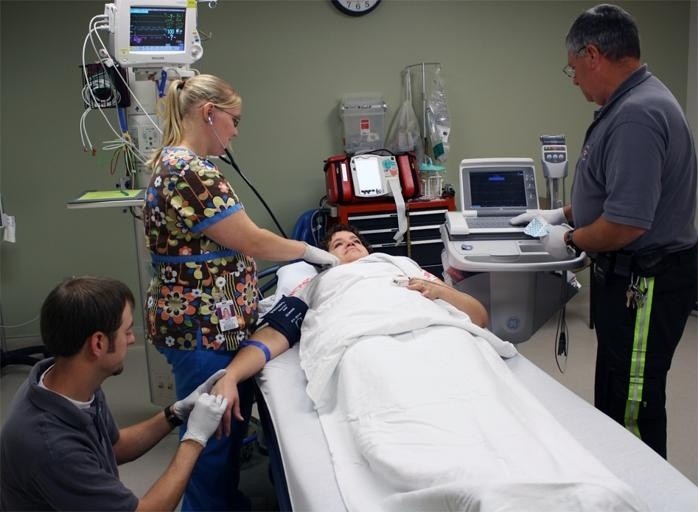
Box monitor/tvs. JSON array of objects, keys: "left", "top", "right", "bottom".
[
  {"left": 107, "top": 0, "right": 198, "bottom": 65},
  {"left": 459, "top": 165, "right": 540, "bottom": 217}
]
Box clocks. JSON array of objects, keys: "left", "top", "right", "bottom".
[{"left": 330, "top": 0, "right": 382, "bottom": 17}]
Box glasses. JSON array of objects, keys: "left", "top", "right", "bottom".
[
  {"left": 214, "top": 105, "right": 240, "bottom": 128},
  {"left": 562, "top": 45, "right": 585, "bottom": 78}
]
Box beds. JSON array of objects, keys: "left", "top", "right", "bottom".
[{"left": 250, "top": 256, "right": 698, "bottom": 512}]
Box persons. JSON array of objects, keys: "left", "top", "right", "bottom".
[
  {"left": 505, "top": 2, "right": 698, "bottom": 461},
  {"left": 140, "top": 75, "right": 343, "bottom": 511},
  {"left": 222, "top": 307, "right": 231, "bottom": 318},
  {"left": 209, "top": 223, "right": 490, "bottom": 443},
  {"left": 0, "top": 275, "right": 228, "bottom": 512}
]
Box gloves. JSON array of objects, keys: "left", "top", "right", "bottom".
[
  {"left": 302, "top": 242, "right": 340, "bottom": 267},
  {"left": 174, "top": 369, "right": 228, "bottom": 448},
  {"left": 509, "top": 207, "right": 577, "bottom": 261}
]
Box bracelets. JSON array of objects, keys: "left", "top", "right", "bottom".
[{"left": 163, "top": 405, "right": 182, "bottom": 427}]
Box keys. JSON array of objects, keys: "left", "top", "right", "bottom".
[{"left": 625, "top": 288, "right": 643, "bottom": 309}]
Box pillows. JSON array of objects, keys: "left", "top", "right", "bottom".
[{"left": 272, "top": 262, "right": 318, "bottom": 310}]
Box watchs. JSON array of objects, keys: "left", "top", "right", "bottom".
[{"left": 566, "top": 229, "right": 582, "bottom": 260}]
